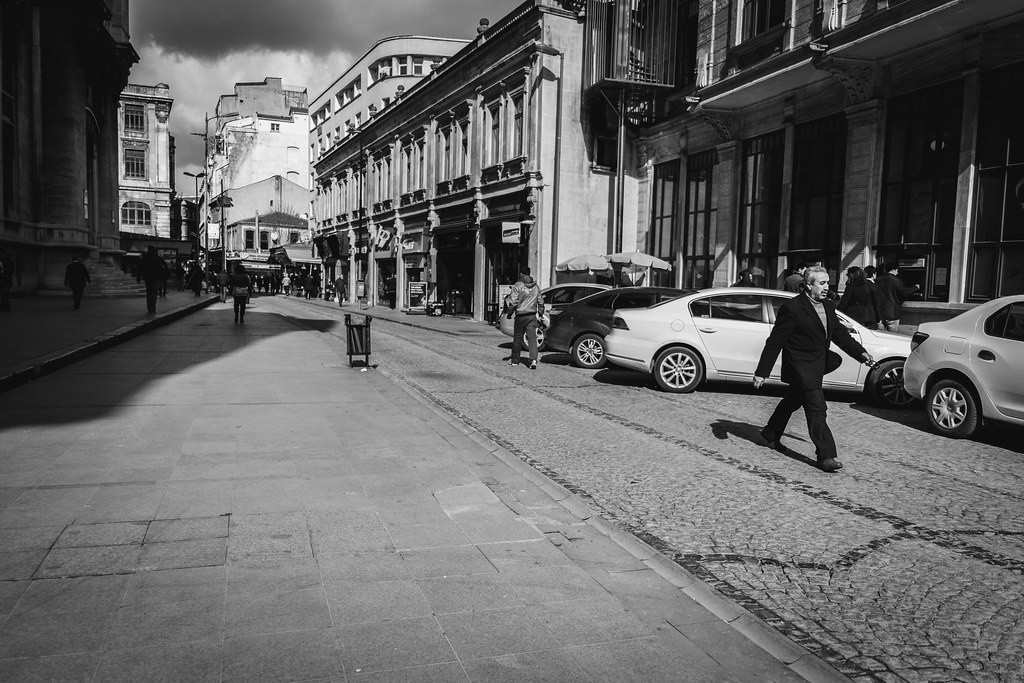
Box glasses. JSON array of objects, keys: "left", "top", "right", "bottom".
[{"left": 865, "top": 356, "right": 880, "bottom": 372}]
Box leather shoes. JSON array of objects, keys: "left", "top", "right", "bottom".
[
  {"left": 760, "top": 426, "right": 786, "bottom": 451},
  {"left": 816, "top": 455, "right": 843, "bottom": 470}
]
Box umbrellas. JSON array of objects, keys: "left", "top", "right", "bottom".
[
  {"left": 555, "top": 253, "right": 613, "bottom": 283},
  {"left": 604, "top": 250, "right": 671, "bottom": 286}
]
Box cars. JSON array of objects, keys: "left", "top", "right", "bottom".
[
  {"left": 902, "top": 294, "right": 1024, "bottom": 449},
  {"left": 545, "top": 287, "right": 694, "bottom": 369},
  {"left": 601, "top": 287, "right": 918, "bottom": 411},
  {"left": 502, "top": 283, "right": 612, "bottom": 352}
]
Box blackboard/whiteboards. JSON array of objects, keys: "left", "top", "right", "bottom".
[{"left": 409, "top": 282, "right": 427, "bottom": 309}]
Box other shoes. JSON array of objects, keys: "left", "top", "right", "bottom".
[
  {"left": 235, "top": 315, "right": 238, "bottom": 321},
  {"left": 240, "top": 319, "right": 245, "bottom": 323}
]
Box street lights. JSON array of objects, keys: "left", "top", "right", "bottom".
[
  {"left": 532, "top": 40, "right": 566, "bottom": 286},
  {"left": 182, "top": 172, "right": 207, "bottom": 265},
  {"left": 190, "top": 111, "right": 239, "bottom": 294},
  {"left": 347, "top": 129, "right": 366, "bottom": 282}
]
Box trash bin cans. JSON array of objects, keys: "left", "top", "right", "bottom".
[
  {"left": 487, "top": 303, "right": 498, "bottom": 325},
  {"left": 344, "top": 313, "right": 373, "bottom": 368},
  {"left": 357, "top": 279, "right": 364, "bottom": 298}
]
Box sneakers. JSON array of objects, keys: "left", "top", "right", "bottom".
[
  {"left": 508, "top": 358, "right": 518, "bottom": 366},
  {"left": 528, "top": 359, "right": 537, "bottom": 370}
]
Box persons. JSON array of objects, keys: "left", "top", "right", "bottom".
[
  {"left": 305, "top": 274, "right": 314, "bottom": 299},
  {"left": 0, "top": 254, "right": 15, "bottom": 310},
  {"left": 137, "top": 245, "right": 170, "bottom": 312},
  {"left": 730, "top": 270, "right": 756, "bottom": 287},
  {"left": 335, "top": 274, "right": 348, "bottom": 307},
  {"left": 281, "top": 273, "right": 291, "bottom": 296},
  {"left": 838, "top": 263, "right": 920, "bottom": 334},
  {"left": 257, "top": 274, "right": 281, "bottom": 294},
  {"left": 175, "top": 260, "right": 231, "bottom": 303},
  {"left": 64, "top": 258, "right": 91, "bottom": 309},
  {"left": 506, "top": 266, "right": 544, "bottom": 369},
  {"left": 752, "top": 267, "right": 873, "bottom": 471},
  {"left": 783, "top": 264, "right": 807, "bottom": 292},
  {"left": 231, "top": 265, "right": 256, "bottom": 323}
]
